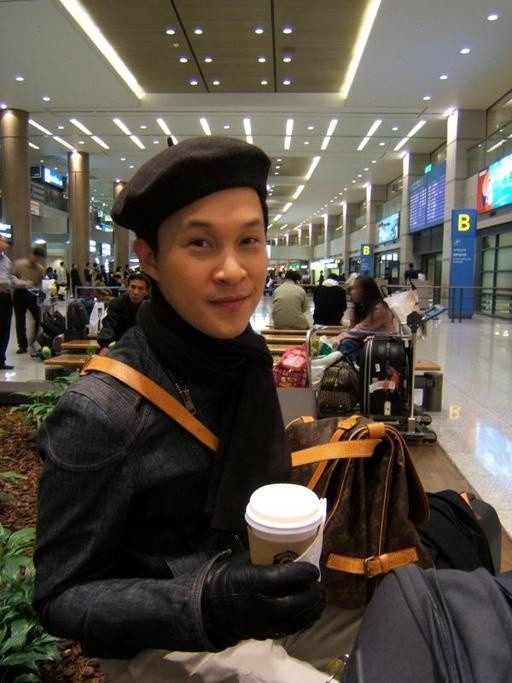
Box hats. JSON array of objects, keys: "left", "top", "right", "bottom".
[{"left": 110, "top": 136, "right": 272, "bottom": 231}]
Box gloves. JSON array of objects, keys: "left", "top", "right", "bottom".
[{"left": 206, "top": 548, "right": 326, "bottom": 641}]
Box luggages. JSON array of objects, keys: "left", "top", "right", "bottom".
[
  {"left": 362, "top": 335, "right": 411, "bottom": 432},
  {"left": 426, "top": 487, "right": 501, "bottom": 575},
  {"left": 339, "top": 561, "right": 512, "bottom": 682}
]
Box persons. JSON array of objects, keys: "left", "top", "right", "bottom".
[
  {"left": 263, "top": 262, "right": 425, "bottom": 418},
  {"left": 31, "top": 134, "right": 366, "bottom": 683},
  {"left": 9, "top": 245, "right": 47, "bottom": 354},
  {"left": 95, "top": 274, "right": 152, "bottom": 358},
  {"left": 32, "top": 261, "right": 150, "bottom": 301},
  {"left": 0, "top": 235, "right": 33, "bottom": 370}
]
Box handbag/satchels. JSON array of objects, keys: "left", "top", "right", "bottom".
[
  {"left": 316, "top": 360, "right": 360, "bottom": 419},
  {"left": 274, "top": 347, "right": 308, "bottom": 387},
  {"left": 284, "top": 413, "right": 432, "bottom": 609}
]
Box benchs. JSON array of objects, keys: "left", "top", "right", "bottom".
[{"left": 272, "top": 284, "right": 317, "bottom": 294}]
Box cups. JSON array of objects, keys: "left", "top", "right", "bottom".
[{"left": 244, "top": 483, "right": 326, "bottom": 565}]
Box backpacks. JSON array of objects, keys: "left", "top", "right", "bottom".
[{"left": 36, "top": 296, "right": 95, "bottom": 351}]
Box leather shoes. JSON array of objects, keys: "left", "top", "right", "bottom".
[
  {"left": 0, "top": 365, "right": 15, "bottom": 370},
  {"left": 16, "top": 349, "right": 28, "bottom": 354}
]
